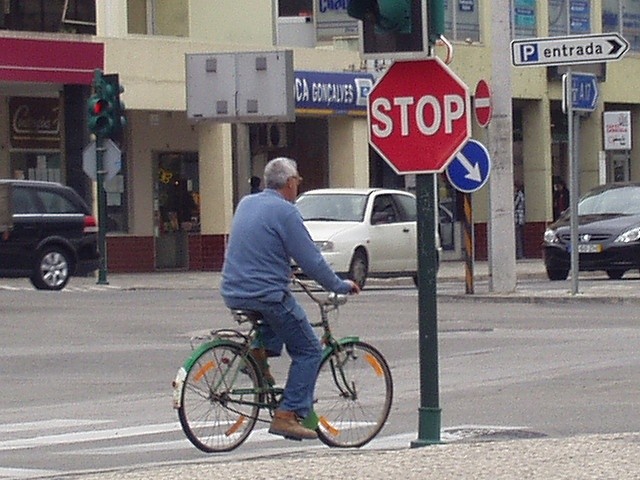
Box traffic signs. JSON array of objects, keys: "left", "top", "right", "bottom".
[
  {"left": 566, "top": 72, "right": 598, "bottom": 112},
  {"left": 509, "top": 32, "right": 629, "bottom": 67}
]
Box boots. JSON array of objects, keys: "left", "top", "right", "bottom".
[
  {"left": 239, "top": 348, "right": 275, "bottom": 385},
  {"left": 268, "top": 410, "right": 318, "bottom": 439}
]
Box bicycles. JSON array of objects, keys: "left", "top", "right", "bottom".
[{"left": 173, "top": 279, "right": 393, "bottom": 452}]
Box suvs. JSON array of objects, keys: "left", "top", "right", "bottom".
[{"left": 0, "top": 179, "right": 100, "bottom": 291}]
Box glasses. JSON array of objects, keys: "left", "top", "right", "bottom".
[{"left": 287, "top": 175, "right": 304, "bottom": 185}]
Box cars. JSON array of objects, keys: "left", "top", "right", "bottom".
[
  {"left": 540, "top": 182, "right": 640, "bottom": 279},
  {"left": 289, "top": 188, "right": 440, "bottom": 289}
]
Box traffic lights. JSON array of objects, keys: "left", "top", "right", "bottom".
[
  {"left": 102, "top": 73, "right": 127, "bottom": 142},
  {"left": 87, "top": 95, "right": 112, "bottom": 135},
  {"left": 346, "top": 0, "right": 423, "bottom": 53}
]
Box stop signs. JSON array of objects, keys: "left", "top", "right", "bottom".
[
  {"left": 366, "top": 57, "right": 470, "bottom": 176},
  {"left": 473, "top": 80, "right": 492, "bottom": 127}
]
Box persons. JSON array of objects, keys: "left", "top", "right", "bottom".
[
  {"left": 514, "top": 184, "right": 525, "bottom": 259},
  {"left": 219, "top": 157, "right": 360, "bottom": 440},
  {"left": 251, "top": 176, "right": 262, "bottom": 193},
  {"left": 553, "top": 175, "right": 569, "bottom": 221}
]
